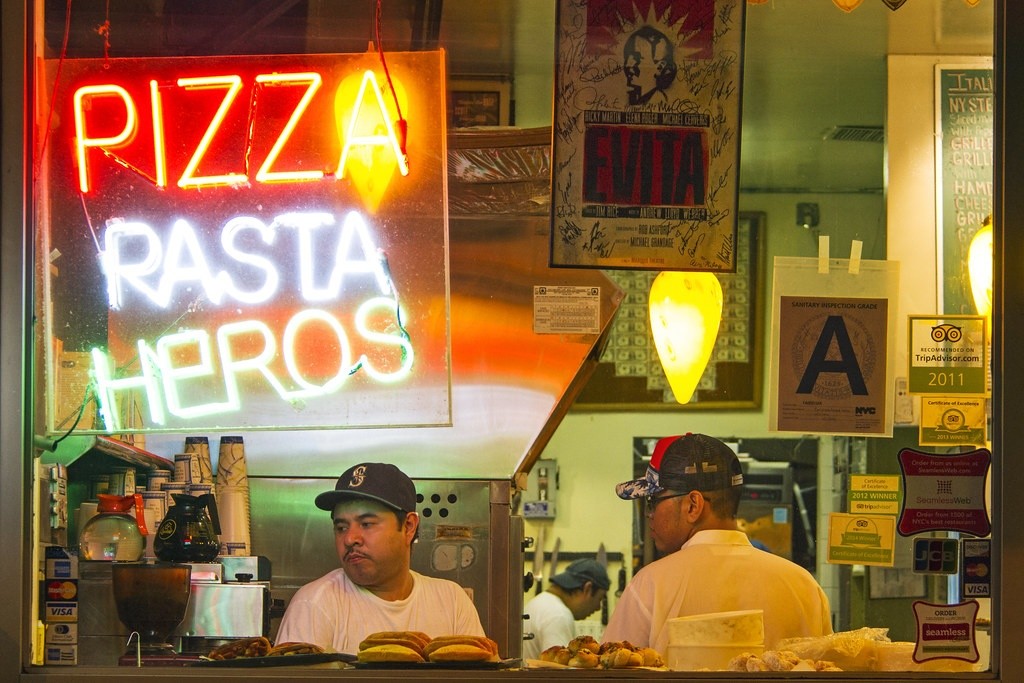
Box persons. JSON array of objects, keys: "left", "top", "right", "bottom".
[
  {"left": 522, "top": 557, "right": 612, "bottom": 659},
  {"left": 604, "top": 432, "right": 833, "bottom": 653},
  {"left": 272, "top": 462, "right": 486, "bottom": 658}
]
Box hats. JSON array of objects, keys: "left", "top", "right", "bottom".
[
  {"left": 616, "top": 432, "right": 744, "bottom": 500},
  {"left": 315, "top": 463, "right": 416, "bottom": 513},
  {"left": 751, "top": 542, "right": 769, "bottom": 551},
  {"left": 549, "top": 558, "right": 610, "bottom": 591}
]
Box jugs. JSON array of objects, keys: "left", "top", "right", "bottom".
[
  {"left": 80, "top": 493, "right": 148, "bottom": 562},
  {"left": 153, "top": 493, "right": 222, "bottom": 563}
]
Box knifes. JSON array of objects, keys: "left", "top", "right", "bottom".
[
  {"left": 549, "top": 537, "right": 560, "bottom": 577},
  {"left": 532, "top": 523, "right": 544, "bottom": 596},
  {"left": 597, "top": 543, "right": 608, "bottom": 625}
]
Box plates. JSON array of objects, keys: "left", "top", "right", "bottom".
[
  {"left": 348, "top": 658, "right": 523, "bottom": 670},
  {"left": 199, "top": 652, "right": 358, "bottom": 668}
]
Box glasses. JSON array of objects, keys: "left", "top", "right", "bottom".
[{"left": 646, "top": 492, "right": 711, "bottom": 513}]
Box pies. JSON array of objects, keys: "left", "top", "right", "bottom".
[
  {"left": 353, "top": 631, "right": 500, "bottom": 661},
  {"left": 207, "top": 636, "right": 323, "bottom": 659}
]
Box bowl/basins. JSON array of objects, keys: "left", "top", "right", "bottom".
[
  {"left": 666, "top": 610, "right": 765, "bottom": 645},
  {"left": 668, "top": 644, "right": 764, "bottom": 671}
]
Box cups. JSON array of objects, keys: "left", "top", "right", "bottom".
[{"left": 74, "top": 436, "right": 251, "bottom": 558}]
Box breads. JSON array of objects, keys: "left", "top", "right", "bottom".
[
  {"left": 539, "top": 635, "right": 663, "bottom": 668},
  {"left": 725, "top": 649, "right": 843, "bottom": 671}
]
